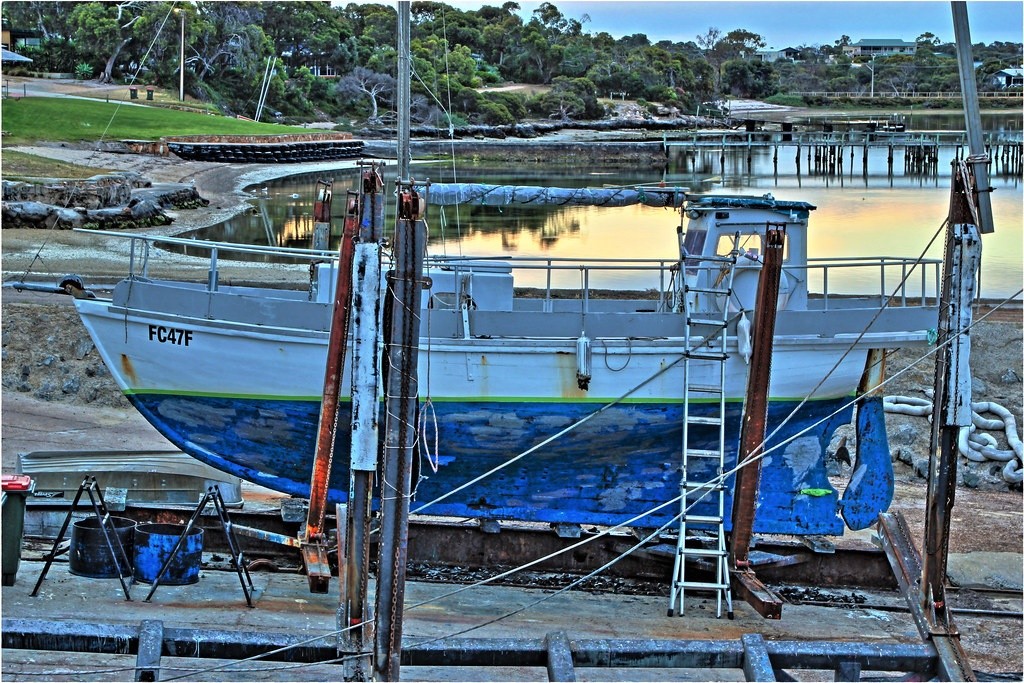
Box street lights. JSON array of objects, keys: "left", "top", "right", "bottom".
[{"left": 174, "top": 6, "right": 186, "bottom": 103}]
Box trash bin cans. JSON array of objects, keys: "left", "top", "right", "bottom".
[{"left": 2, "top": 474, "right": 36, "bottom": 587}]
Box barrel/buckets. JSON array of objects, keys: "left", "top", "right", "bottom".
[
  {"left": 135, "top": 524, "right": 204, "bottom": 585},
  {"left": 68, "top": 516, "right": 138, "bottom": 578}
]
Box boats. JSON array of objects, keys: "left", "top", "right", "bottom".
[{"left": 60, "top": 192, "right": 943, "bottom": 543}]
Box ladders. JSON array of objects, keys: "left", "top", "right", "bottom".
[{"left": 668, "top": 225, "right": 741, "bottom": 620}]
